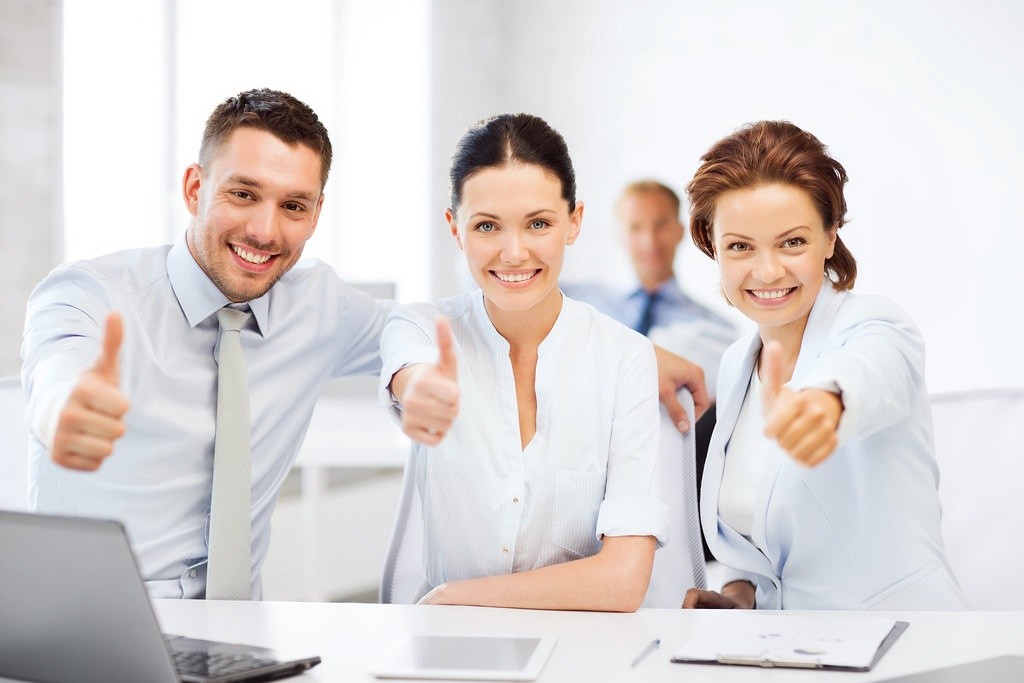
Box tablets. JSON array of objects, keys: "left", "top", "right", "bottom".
[{"left": 375, "top": 630, "right": 557, "bottom": 682}]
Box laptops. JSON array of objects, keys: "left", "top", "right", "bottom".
[{"left": 1, "top": 512, "right": 321, "bottom": 683}]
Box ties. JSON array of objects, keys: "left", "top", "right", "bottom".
[
  {"left": 204, "top": 306, "right": 255, "bottom": 600},
  {"left": 633, "top": 290, "right": 663, "bottom": 337}
]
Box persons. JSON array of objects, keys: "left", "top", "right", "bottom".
[
  {"left": 574, "top": 180, "right": 743, "bottom": 395},
  {"left": 17, "top": 86, "right": 712, "bottom": 598},
  {"left": 677, "top": 119, "right": 946, "bottom": 611},
  {"left": 377, "top": 111, "right": 661, "bottom": 616}
]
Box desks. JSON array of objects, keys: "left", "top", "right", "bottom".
[{"left": 0, "top": 586, "right": 1024, "bottom": 682}]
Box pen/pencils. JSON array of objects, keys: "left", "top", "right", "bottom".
[{"left": 631, "top": 640, "right": 660, "bottom": 669}]
